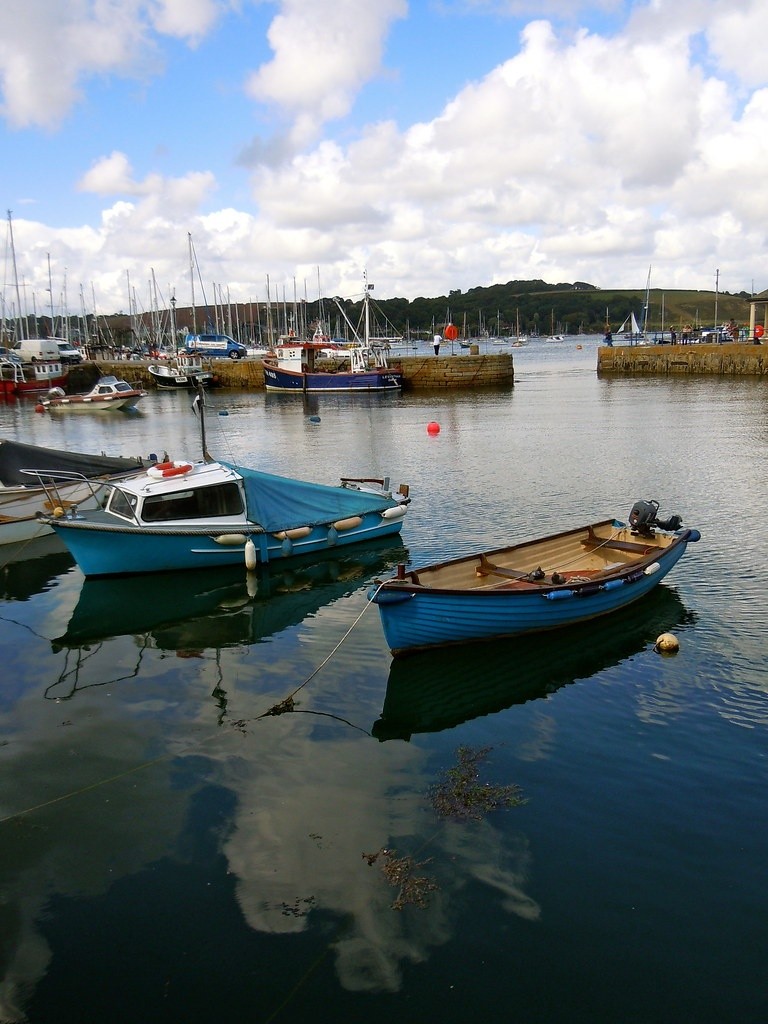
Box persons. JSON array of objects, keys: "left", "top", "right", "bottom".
[
  {"left": 680, "top": 324, "right": 692, "bottom": 345},
  {"left": 433, "top": 333, "right": 442, "bottom": 356},
  {"left": 722, "top": 317, "right": 750, "bottom": 343},
  {"left": 669, "top": 325, "right": 676, "bottom": 345},
  {"left": 605, "top": 323, "right": 613, "bottom": 347}
]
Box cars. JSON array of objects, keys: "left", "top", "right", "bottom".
[{"left": 0, "top": 346, "right": 25, "bottom": 364}]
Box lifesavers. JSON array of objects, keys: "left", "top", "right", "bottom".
[
  {"left": 446, "top": 324, "right": 458, "bottom": 340},
  {"left": 147, "top": 460, "right": 195, "bottom": 479},
  {"left": 754, "top": 325, "right": 764, "bottom": 338}
]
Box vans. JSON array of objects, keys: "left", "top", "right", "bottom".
[
  {"left": 56, "top": 340, "right": 81, "bottom": 364},
  {"left": 9, "top": 339, "right": 61, "bottom": 364},
  {"left": 186, "top": 334, "right": 248, "bottom": 359}
]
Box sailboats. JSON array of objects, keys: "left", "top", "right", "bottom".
[{"left": 0, "top": 207, "right": 756, "bottom": 405}]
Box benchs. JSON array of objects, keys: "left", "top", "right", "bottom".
[
  {"left": 475, "top": 564, "right": 566, "bottom": 586},
  {"left": 677, "top": 337, "right": 703, "bottom": 344},
  {"left": 581, "top": 536, "right": 665, "bottom": 556}
]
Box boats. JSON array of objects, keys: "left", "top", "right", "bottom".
[
  {"left": 49, "top": 533, "right": 409, "bottom": 655},
  {"left": 371, "top": 581, "right": 687, "bottom": 743},
  {"left": 365, "top": 498, "right": 701, "bottom": 656},
  {"left": 34, "top": 375, "right": 149, "bottom": 413},
  {"left": 18, "top": 384, "right": 411, "bottom": 580}
]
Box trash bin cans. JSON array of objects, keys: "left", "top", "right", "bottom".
[{"left": 470, "top": 345, "right": 479, "bottom": 355}]
